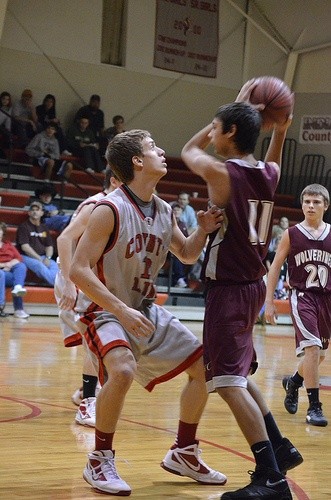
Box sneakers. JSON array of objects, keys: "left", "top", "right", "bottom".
[
  {"left": 248, "top": 436, "right": 303, "bottom": 481},
  {"left": 14, "top": 310, "right": 29, "bottom": 318},
  {"left": 10, "top": 288, "right": 26, "bottom": 298},
  {"left": 306, "top": 403, "right": 327, "bottom": 426},
  {"left": 74, "top": 396, "right": 97, "bottom": 428},
  {"left": 221, "top": 468, "right": 293, "bottom": 500},
  {"left": 282, "top": 376, "right": 298, "bottom": 414},
  {"left": 160, "top": 445, "right": 227, "bottom": 485},
  {"left": 72, "top": 387, "right": 98, "bottom": 407},
  {"left": 82, "top": 449, "right": 131, "bottom": 495}
]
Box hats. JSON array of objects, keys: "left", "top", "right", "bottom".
[
  {"left": 37, "top": 183, "right": 56, "bottom": 199},
  {"left": 31, "top": 201, "right": 42, "bottom": 211},
  {"left": 45, "top": 120, "right": 61, "bottom": 134},
  {"left": 171, "top": 203, "right": 184, "bottom": 210}
]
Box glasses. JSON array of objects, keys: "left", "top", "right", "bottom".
[{"left": 23, "top": 94, "right": 32, "bottom": 98}]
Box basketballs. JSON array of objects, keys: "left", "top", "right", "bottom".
[{"left": 246, "top": 76, "right": 295, "bottom": 129}]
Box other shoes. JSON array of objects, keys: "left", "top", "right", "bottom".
[
  {"left": 277, "top": 291, "right": 288, "bottom": 299},
  {"left": 62, "top": 150, "right": 72, "bottom": 156},
  {"left": 45, "top": 178, "right": 50, "bottom": 183},
  {"left": 0, "top": 310, "right": 6, "bottom": 317},
  {"left": 64, "top": 180, "right": 68, "bottom": 184},
  {"left": 85, "top": 167, "right": 95, "bottom": 174},
  {"left": 174, "top": 278, "right": 188, "bottom": 288}
]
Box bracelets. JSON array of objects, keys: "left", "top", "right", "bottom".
[{"left": 46, "top": 256, "right": 51, "bottom": 260}]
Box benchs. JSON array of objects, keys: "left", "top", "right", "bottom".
[{"left": 0, "top": 138, "right": 306, "bottom": 317}]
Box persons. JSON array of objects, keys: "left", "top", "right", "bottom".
[
  {"left": 36, "top": 94, "right": 72, "bottom": 155},
  {"left": 12, "top": 90, "right": 39, "bottom": 150},
  {"left": 264, "top": 216, "right": 289, "bottom": 282},
  {"left": 0, "top": 221, "right": 30, "bottom": 319},
  {"left": 0, "top": 91, "right": 13, "bottom": 149},
  {"left": 74, "top": 94, "right": 105, "bottom": 132},
  {"left": 264, "top": 184, "right": 331, "bottom": 426},
  {"left": 54, "top": 163, "right": 125, "bottom": 427},
  {"left": 26, "top": 122, "right": 73, "bottom": 183},
  {"left": 169, "top": 193, "right": 199, "bottom": 288},
  {"left": 17, "top": 201, "right": 60, "bottom": 287},
  {"left": 68, "top": 129, "right": 227, "bottom": 495},
  {"left": 36, "top": 184, "right": 71, "bottom": 231},
  {"left": 104, "top": 115, "right": 126, "bottom": 144},
  {"left": 181, "top": 77, "right": 304, "bottom": 500},
  {"left": 68, "top": 117, "right": 106, "bottom": 174}
]
